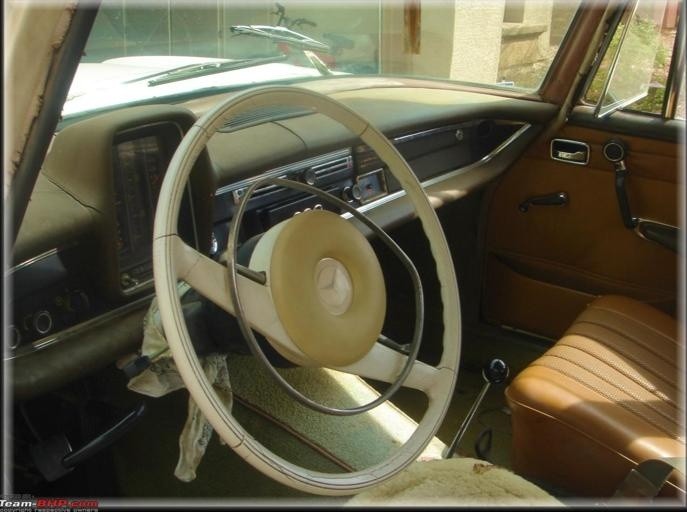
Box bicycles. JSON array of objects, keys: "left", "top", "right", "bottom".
[{"left": 252, "top": 1, "right": 375, "bottom": 72}]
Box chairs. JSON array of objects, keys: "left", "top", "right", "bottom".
[
  {"left": 505, "top": 292, "right": 687, "bottom": 499},
  {"left": 340, "top": 458, "right": 561, "bottom": 505}
]
